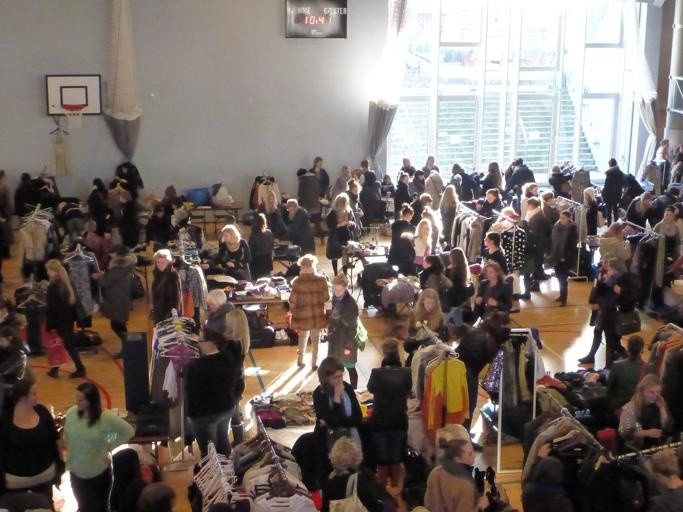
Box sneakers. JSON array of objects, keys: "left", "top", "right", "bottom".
[
  {"left": 47, "top": 370, "right": 58, "bottom": 378},
  {"left": 579, "top": 355, "right": 595, "bottom": 362},
  {"left": 520, "top": 272, "right": 550, "bottom": 299},
  {"left": 556, "top": 296, "right": 566, "bottom": 307},
  {"left": 70, "top": 370, "right": 85, "bottom": 378}
]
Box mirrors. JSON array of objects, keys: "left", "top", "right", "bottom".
[{"left": 495, "top": 352, "right": 536, "bottom": 475}]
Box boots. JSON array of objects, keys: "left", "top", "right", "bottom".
[{"left": 230, "top": 415, "right": 246, "bottom": 448}]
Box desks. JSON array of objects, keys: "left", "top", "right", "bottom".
[
  {"left": 251, "top": 387, "right": 425, "bottom": 461},
  {"left": 124, "top": 433, "right": 170, "bottom": 475},
  {"left": 227, "top": 278, "right": 333, "bottom": 342},
  {"left": 188, "top": 201, "right": 244, "bottom": 234},
  {"left": 570, "top": 383, "right": 608, "bottom": 417}
]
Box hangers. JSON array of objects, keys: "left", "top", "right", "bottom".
[
  {"left": 261, "top": 177, "right": 274, "bottom": 186},
  {"left": 636, "top": 226, "right": 659, "bottom": 241},
  {"left": 15, "top": 185, "right": 155, "bottom": 320},
  {"left": 535, "top": 394, "right": 683, "bottom": 489},
  {"left": 166, "top": 231, "right": 198, "bottom": 259},
  {"left": 417, "top": 335, "right": 461, "bottom": 370},
  {"left": 156, "top": 315, "right": 202, "bottom": 360},
  {"left": 191, "top": 422, "right": 314, "bottom": 511},
  {"left": 48, "top": 126, "right": 71, "bottom": 136},
  {"left": 510, "top": 332, "right": 526, "bottom": 354}
]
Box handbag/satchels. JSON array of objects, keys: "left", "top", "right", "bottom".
[
  {"left": 355, "top": 316, "right": 368, "bottom": 351},
  {"left": 615, "top": 312, "right": 640, "bottom": 335},
  {"left": 329, "top": 494, "right": 370, "bottom": 512},
  {"left": 183, "top": 188, "right": 210, "bottom": 206},
  {"left": 132, "top": 276, "right": 145, "bottom": 299},
  {"left": 209, "top": 184, "right": 233, "bottom": 208},
  {"left": 45, "top": 343, "right": 68, "bottom": 367}
]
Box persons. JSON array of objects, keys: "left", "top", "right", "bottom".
[
  {"left": 671, "top": 145, "right": 683, "bottom": 183},
  {"left": 655, "top": 139, "right": 670, "bottom": 164},
  {"left": 0, "top": 156, "right": 683, "bottom": 512}
]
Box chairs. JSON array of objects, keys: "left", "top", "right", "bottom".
[{"left": 375, "top": 274, "right": 422, "bottom": 319}]
